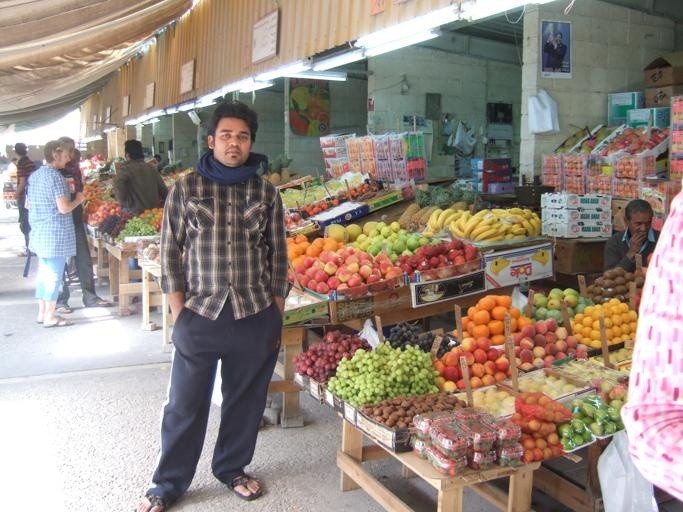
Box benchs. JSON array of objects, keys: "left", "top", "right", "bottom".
[{"left": 332, "top": 408, "right": 542, "bottom": 512}]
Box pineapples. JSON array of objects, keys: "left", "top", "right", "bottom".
[
  {"left": 263, "top": 155, "right": 293, "bottom": 185},
  {"left": 399, "top": 190, "right": 473, "bottom": 233}
]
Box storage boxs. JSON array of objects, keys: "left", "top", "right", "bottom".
[
  {"left": 545, "top": 236, "right": 609, "bottom": 277},
  {"left": 541, "top": 222, "right": 613, "bottom": 239},
  {"left": 645, "top": 85, "right": 682, "bottom": 108},
  {"left": 325, "top": 158, "right": 349, "bottom": 168},
  {"left": 319, "top": 133, "right": 355, "bottom": 148},
  {"left": 609, "top": 92, "right": 645, "bottom": 127},
  {"left": 321, "top": 147, "right": 346, "bottom": 158},
  {"left": 540, "top": 208, "right": 611, "bottom": 224},
  {"left": 625, "top": 107, "right": 670, "bottom": 129},
  {"left": 643, "top": 56, "right": 682, "bottom": 88},
  {"left": 325, "top": 167, "right": 348, "bottom": 179},
  {"left": 539, "top": 193, "right": 613, "bottom": 211},
  {"left": 481, "top": 241, "right": 552, "bottom": 290}
]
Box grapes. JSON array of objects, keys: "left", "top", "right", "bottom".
[
  {"left": 390, "top": 321, "right": 460, "bottom": 358},
  {"left": 328, "top": 341, "right": 438, "bottom": 409}
]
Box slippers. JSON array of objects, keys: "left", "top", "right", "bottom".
[
  {"left": 36, "top": 321, "right": 45, "bottom": 324},
  {"left": 44, "top": 316, "right": 70, "bottom": 327}
]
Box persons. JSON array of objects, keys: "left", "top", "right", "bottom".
[
  {"left": 5, "top": 157, "right": 19, "bottom": 181},
  {"left": 132, "top": 97, "right": 287, "bottom": 512},
  {"left": 25, "top": 139, "right": 86, "bottom": 328},
  {"left": 621, "top": 188, "right": 683, "bottom": 512},
  {"left": 602, "top": 198, "right": 660, "bottom": 276},
  {"left": 55, "top": 134, "right": 112, "bottom": 314},
  {"left": 10, "top": 141, "right": 36, "bottom": 258},
  {"left": 68, "top": 147, "right": 82, "bottom": 169},
  {"left": 113, "top": 136, "right": 168, "bottom": 214}
]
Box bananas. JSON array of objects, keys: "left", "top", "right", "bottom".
[{"left": 422, "top": 208, "right": 541, "bottom": 242}]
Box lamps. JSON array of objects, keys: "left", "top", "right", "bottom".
[
  {"left": 240, "top": 80, "right": 276, "bottom": 94},
  {"left": 284, "top": 70, "right": 348, "bottom": 81},
  {"left": 254, "top": 57, "right": 315, "bottom": 82},
  {"left": 313, "top": 47, "right": 366, "bottom": 72}
]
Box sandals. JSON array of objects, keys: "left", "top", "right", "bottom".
[
  {"left": 133, "top": 494, "right": 172, "bottom": 512},
  {"left": 220, "top": 473, "right": 263, "bottom": 501}
]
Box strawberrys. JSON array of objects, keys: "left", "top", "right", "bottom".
[{"left": 410, "top": 407, "right": 523, "bottom": 476}]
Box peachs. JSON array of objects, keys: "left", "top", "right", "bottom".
[
  {"left": 514, "top": 319, "right": 588, "bottom": 371},
  {"left": 435, "top": 337, "right": 512, "bottom": 393},
  {"left": 289, "top": 247, "right": 402, "bottom": 297}
]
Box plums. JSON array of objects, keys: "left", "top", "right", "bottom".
[{"left": 293, "top": 331, "right": 372, "bottom": 383}]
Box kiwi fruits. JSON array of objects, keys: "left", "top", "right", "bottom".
[{"left": 586, "top": 268, "right": 644, "bottom": 314}]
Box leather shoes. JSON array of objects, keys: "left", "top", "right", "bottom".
[
  {"left": 57, "top": 307, "right": 70, "bottom": 314},
  {"left": 86, "top": 297, "right": 110, "bottom": 307}
]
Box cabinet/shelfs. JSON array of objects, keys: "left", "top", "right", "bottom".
[
  {"left": 103, "top": 243, "right": 144, "bottom": 316},
  {"left": 137, "top": 257, "right": 171, "bottom": 351},
  {"left": 329, "top": 284, "right": 522, "bottom": 331},
  {"left": 533, "top": 438, "right": 677, "bottom": 512},
  {"left": 86, "top": 238, "right": 109, "bottom": 286},
  {"left": 264, "top": 324, "right": 303, "bottom": 427}
]
